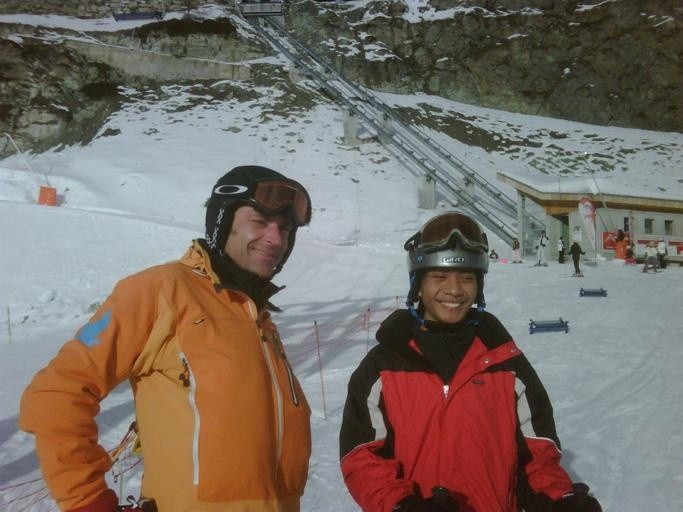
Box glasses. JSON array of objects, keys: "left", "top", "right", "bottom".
[
  {"left": 416, "top": 212, "right": 489, "bottom": 252},
  {"left": 251, "top": 177, "right": 311, "bottom": 227}
]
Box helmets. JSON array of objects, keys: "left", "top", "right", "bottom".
[
  {"left": 407, "top": 213, "right": 489, "bottom": 273},
  {"left": 206, "top": 165, "right": 298, "bottom": 272}
]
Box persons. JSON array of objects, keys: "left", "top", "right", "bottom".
[
  {"left": 338, "top": 210, "right": 582, "bottom": 512},
  {"left": 18, "top": 164, "right": 311, "bottom": 512},
  {"left": 488, "top": 230, "right": 668, "bottom": 277}
]
